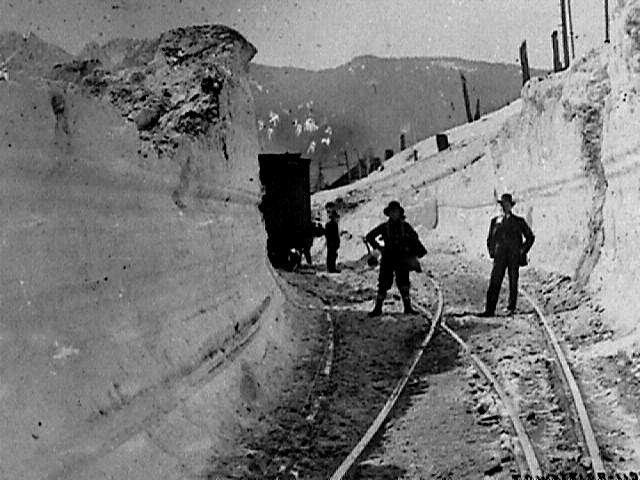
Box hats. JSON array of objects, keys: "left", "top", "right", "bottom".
[
  {"left": 384, "top": 201, "right": 404, "bottom": 216},
  {"left": 497, "top": 194, "right": 514, "bottom": 206},
  {"left": 329, "top": 210, "right": 339, "bottom": 219}
]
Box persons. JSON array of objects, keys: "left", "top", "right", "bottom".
[
  {"left": 323, "top": 208, "right": 344, "bottom": 273},
  {"left": 477, "top": 194, "right": 537, "bottom": 314},
  {"left": 366, "top": 199, "right": 426, "bottom": 315}
]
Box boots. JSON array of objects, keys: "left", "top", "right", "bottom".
[
  {"left": 368, "top": 299, "right": 381, "bottom": 318},
  {"left": 403, "top": 298, "right": 419, "bottom": 315}
]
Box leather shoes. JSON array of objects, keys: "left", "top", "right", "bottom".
[
  {"left": 329, "top": 268, "right": 342, "bottom": 273},
  {"left": 477, "top": 309, "right": 494, "bottom": 317},
  {"left": 498, "top": 308, "right": 512, "bottom": 316}
]
